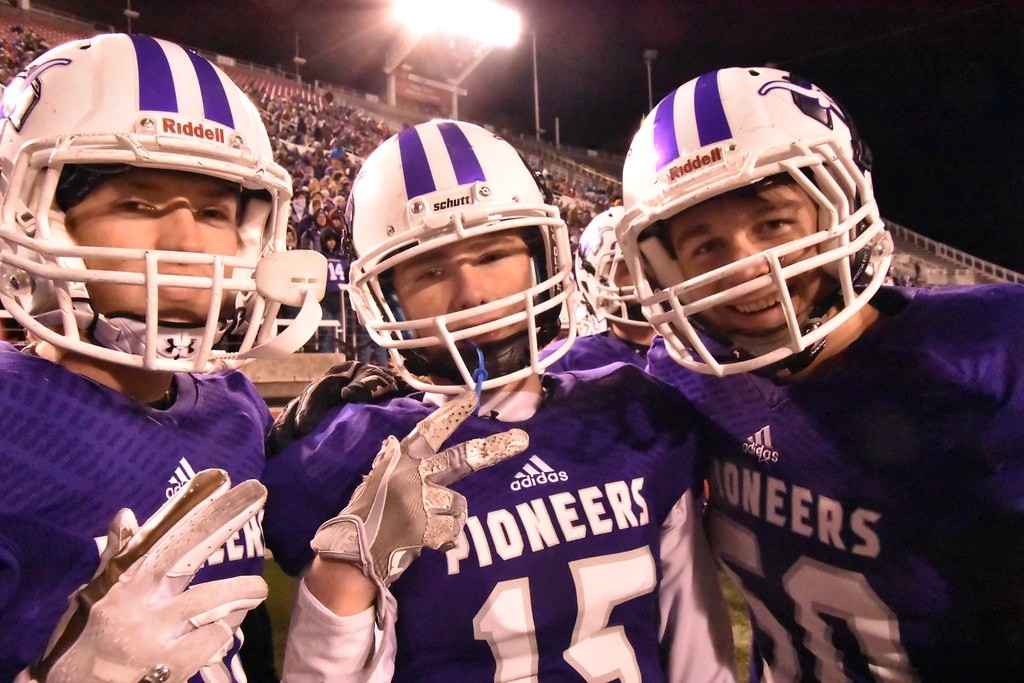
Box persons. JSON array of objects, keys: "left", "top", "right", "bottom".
[
  {"left": 262, "top": 118, "right": 740, "bottom": 683},
  {"left": 884, "top": 264, "right": 923, "bottom": 289},
  {"left": 266, "top": 65, "right": 1024, "bottom": 683},
  {"left": 0, "top": 18, "right": 51, "bottom": 86},
  {"left": 0, "top": 33, "right": 398, "bottom": 683},
  {"left": 528, "top": 162, "right": 623, "bottom": 250},
  {"left": 243, "top": 80, "right": 392, "bottom": 370},
  {"left": 536, "top": 205, "right": 660, "bottom": 374}
]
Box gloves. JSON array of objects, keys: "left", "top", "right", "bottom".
[
  {"left": 310, "top": 389, "right": 531, "bottom": 627},
  {"left": 13, "top": 467, "right": 270, "bottom": 682}
]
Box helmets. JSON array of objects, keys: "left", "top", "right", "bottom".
[
  {"left": 574, "top": 204, "right": 653, "bottom": 330},
  {"left": 1, "top": 31, "right": 294, "bottom": 376},
  {"left": 614, "top": 66, "right": 896, "bottom": 379},
  {"left": 344, "top": 119, "right": 579, "bottom": 396}
]
